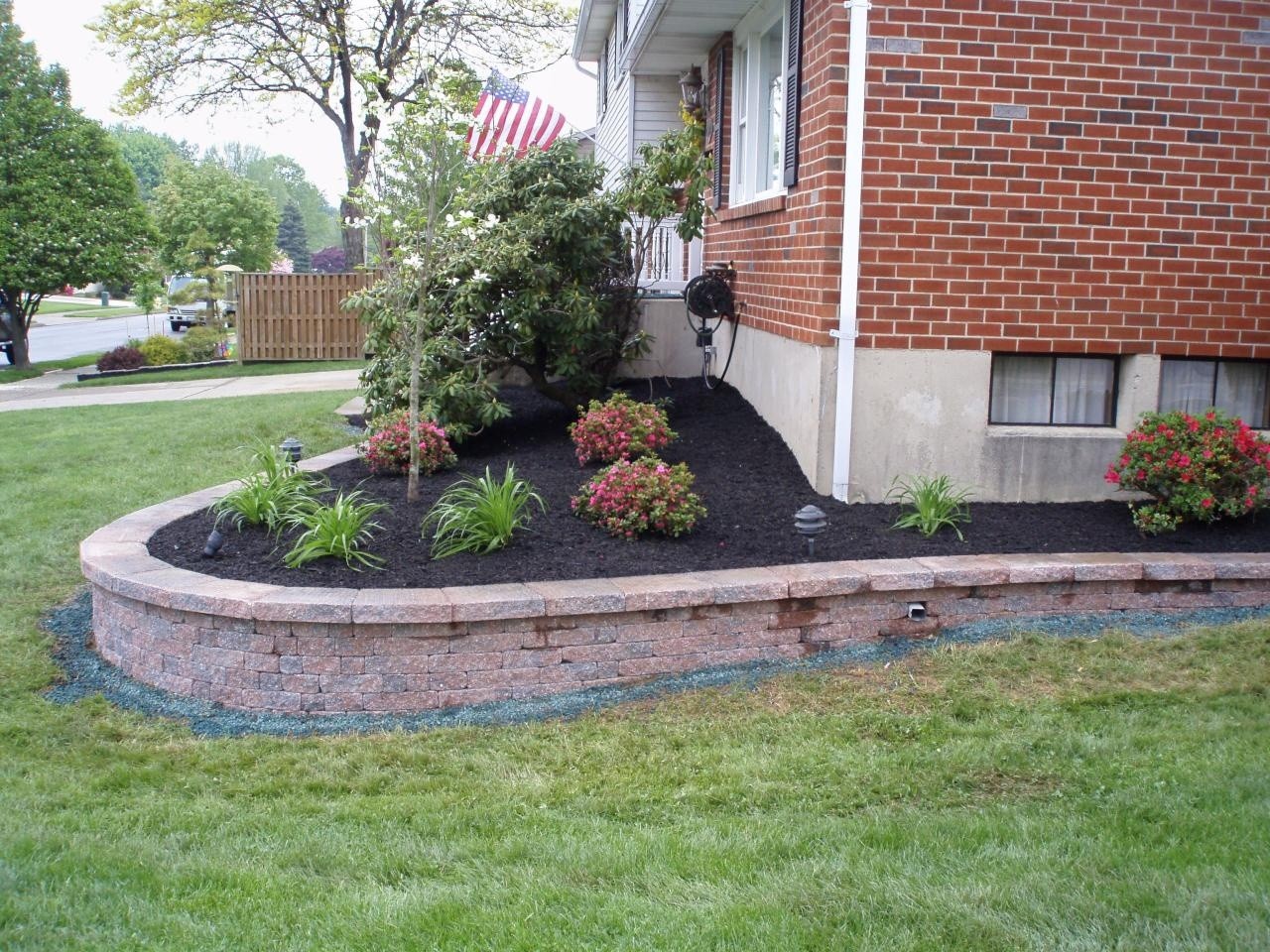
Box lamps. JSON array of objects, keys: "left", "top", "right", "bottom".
[
  {"left": 278, "top": 437, "right": 304, "bottom": 473},
  {"left": 204, "top": 529, "right": 222, "bottom": 556},
  {"left": 794, "top": 504, "right": 829, "bottom": 556},
  {"left": 678, "top": 62, "right": 711, "bottom": 122}
]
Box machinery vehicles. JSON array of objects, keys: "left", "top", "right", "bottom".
[{"left": 163, "top": 264, "right": 244, "bottom": 331}]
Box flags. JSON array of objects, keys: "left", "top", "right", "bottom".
[{"left": 463, "top": 69, "right": 565, "bottom": 169}]
